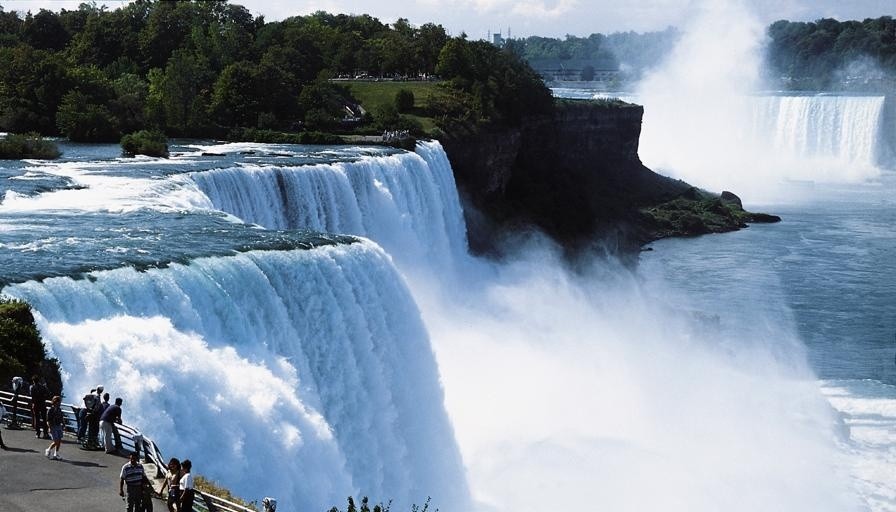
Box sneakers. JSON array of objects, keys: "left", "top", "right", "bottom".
[
  {"left": 46, "top": 452, "right": 62, "bottom": 460},
  {"left": 35, "top": 434, "right": 52, "bottom": 439},
  {"left": 1, "top": 446, "right": 8, "bottom": 449},
  {"left": 77, "top": 436, "right": 116, "bottom": 453}
]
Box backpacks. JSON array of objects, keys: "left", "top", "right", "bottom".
[{"left": 83, "top": 395, "right": 96, "bottom": 412}]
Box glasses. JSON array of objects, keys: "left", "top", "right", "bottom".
[
  {"left": 122, "top": 495, "right": 125, "bottom": 501},
  {"left": 169, "top": 462, "right": 179, "bottom": 465}
]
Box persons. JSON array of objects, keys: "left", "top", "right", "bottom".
[
  {"left": 29, "top": 375, "right": 193, "bottom": 512},
  {"left": 0, "top": 401, "right": 7, "bottom": 449}
]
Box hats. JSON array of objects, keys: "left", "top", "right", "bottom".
[
  {"left": 96, "top": 385, "right": 104, "bottom": 391},
  {"left": 181, "top": 459, "right": 191, "bottom": 468}
]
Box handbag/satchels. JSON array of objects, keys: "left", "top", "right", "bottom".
[{"left": 171, "top": 491, "right": 176, "bottom": 497}]
